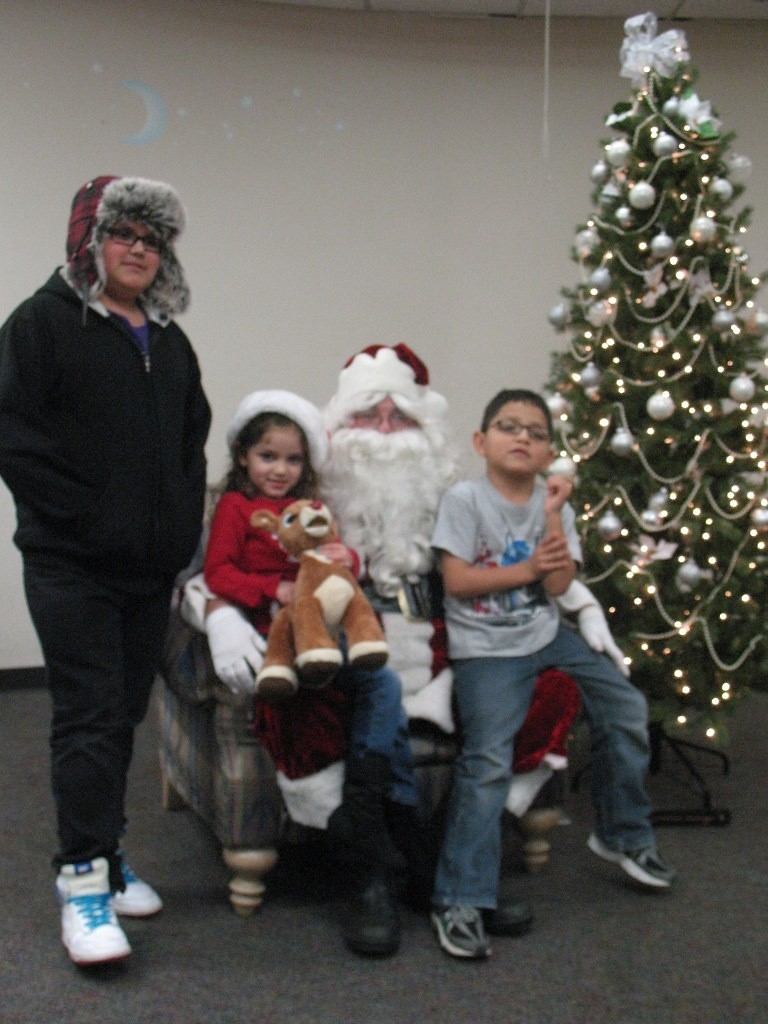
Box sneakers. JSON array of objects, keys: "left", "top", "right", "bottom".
[
  {"left": 428, "top": 906, "right": 492, "bottom": 958},
  {"left": 110, "top": 849, "right": 164, "bottom": 917},
  {"left": 587, "top": 831, "right": 676, "bottom": 888},
  {"left": 55, "top": 856, "right": 132, "bottom": 966}
]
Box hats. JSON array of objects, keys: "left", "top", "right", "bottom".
[
  {"left": 339, "top": 344, "right": 428, "bottom": 399},
  {"left": 227, "top": 391, "right": 329, "bottom": 473},
  {"left": 66, "top": 176, "right": 191, "bottom": 327}
]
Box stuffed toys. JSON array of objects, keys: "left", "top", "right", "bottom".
[{"left": 249, "top": 498, "right": 391, "bottom": 695}]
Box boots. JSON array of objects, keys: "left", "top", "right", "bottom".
[
  {"left": 275, "top": 761, "right": 400, "bottom": 952},
  {"left": 404, "top": 770, "right": 559, "bottom": 934},
  {"left": 326, "top": 750, "right": 409, "bottom": 883}
]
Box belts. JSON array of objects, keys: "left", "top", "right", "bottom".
[{"left": 357, "top": 570, "right": 446, "bottom": 621}]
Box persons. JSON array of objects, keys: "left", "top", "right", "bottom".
[
  {"left": 432, "top": 387, "right": 675, "bottom": 961},
  {"left": 178, "top": 343, "right": 580, "bottom": 962},
  {"left": 0, "top": 176, "right": 212, "bottom": 964},
  {"left": 202, "top": 387, "right": 421, "bottom": 808}
]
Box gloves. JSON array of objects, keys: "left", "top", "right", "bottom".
[
  {"left": 578, "top": 606, "right": 630, "bottom": 677},
  {"left": 206, "top": 606, "right": 267, "bottom": 694}
]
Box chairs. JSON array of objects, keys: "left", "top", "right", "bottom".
[{"left": 157, "top": 482, "right": 563, "bottom": 914}]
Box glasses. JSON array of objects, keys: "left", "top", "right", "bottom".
[
  {"left": 355, "top": 413, "right": 414, "bottom": 428},
  {"left": 487, "top": 418, "right": 550, "bottom": 443},
  {"left": 99, "top": 226, "right": 164, "bottom": 253}
]
